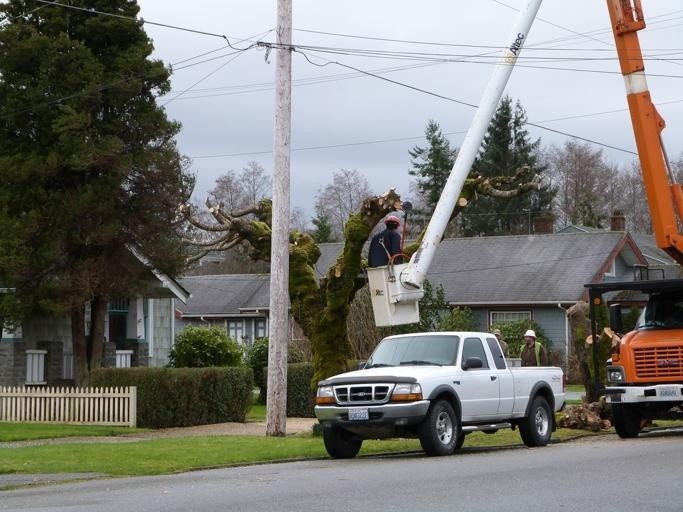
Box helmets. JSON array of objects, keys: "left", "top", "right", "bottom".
[
  {"left": 524, "top": 330, "right": 537, "bottom": 338},
  {"left": 384, "top": 216, "right": 400, "bottom": 226}
]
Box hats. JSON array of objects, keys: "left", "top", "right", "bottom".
[{"left": 493, "top": 329, "right": 501, "bottom": 336}]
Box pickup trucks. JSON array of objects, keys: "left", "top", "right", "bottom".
[{"left": 313, "top": 330, "right": 565, "bottom": 459}]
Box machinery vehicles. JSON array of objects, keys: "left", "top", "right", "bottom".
[{"left": 364, "top": 1, "right": 682, "bottom": 438}]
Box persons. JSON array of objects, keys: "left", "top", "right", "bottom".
[
  {"left": 494, "top": 329, "right": 509, "bottom": 357},
  {"left": 520, "top": 330, "right": 548, "bottom": 366},
  {"left": 368, "top": 216, "right": 401, "bottom": 268}
]
